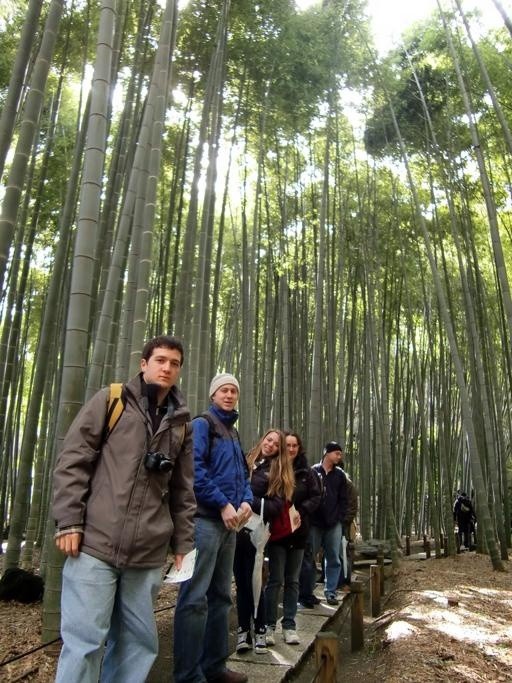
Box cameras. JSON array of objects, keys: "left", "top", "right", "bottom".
[{"left": 143, "top": 452, "right": 174, "bottom": 475}]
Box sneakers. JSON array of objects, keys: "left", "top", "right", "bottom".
[
  {"left": 281, "top": 628, "right": 300, "bottom": 644},
  {"left": 336, "top": 584, "right": 351, "bottom": 593},
  {"left": 254, "top": 628, "right": 267, "bottom": 654},
  {"left": 206, "top": 668, "right": 248, "bottom": 683},
  {"left": 236, "top": 625, "right": 253, "bottom": 652},
  {"left": 296, "top": 604, "right": 313, "bottom": 612},
  {"left": 326, "top": 591, "right": 338, "bottom": 604},
  {"left": 265, "top": 625, "right": 276, "bottom": 645}
]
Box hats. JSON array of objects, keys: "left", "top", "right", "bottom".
[
  {"left": 209, "top": 373, "right": 240, "bottom": 399},
  {"left": 322, "top": 440, "right": 342, "bottom": 457}
]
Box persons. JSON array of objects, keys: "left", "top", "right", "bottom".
[
  {"left": 263, "top": 430, "right": 323, "bottom": 644},
  {"left": 335, "top": 458, "right": 358, "bottom": 594},
  {"left": 310, "top": 440, "right": 347, "bottom": 605},
  {"left": 299, "top": 530, "right": 336, "bottom": 616},
  {"left": 50, "top": 337, "right": 197, "bottom": 683},
  {"left": 233, "top": 429, "right": 295, "bottom": 654},
  {"left": 173, "top": 374, "right": 253, "bottom": 683},
  {"left": 452, "top": 493, "right": 477, "bottom": 554}
]
